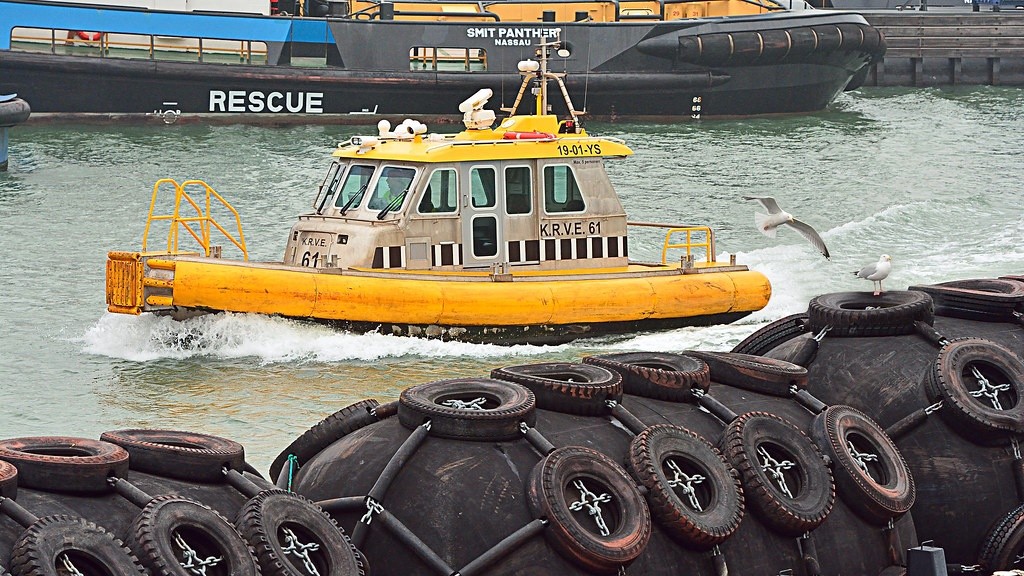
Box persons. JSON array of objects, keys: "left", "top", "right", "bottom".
[{"left": 381, "top": 170, "right": 407, "bottom": 211}]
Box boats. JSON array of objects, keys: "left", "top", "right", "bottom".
[
  {"left": 1, "top": 0, "right": 887, "bottom": 115},
  {"left": 107, "top": 3, "right": 772, "bottom": 345}
]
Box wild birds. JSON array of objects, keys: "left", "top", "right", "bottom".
[
  {"left": 741, "top": 195, "right": 831, "bottom": 262},
  {"left": 850, "top": 254, "right": 892, "bottom": 295}
]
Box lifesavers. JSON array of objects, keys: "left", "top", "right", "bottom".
[
  {"left": 0, "top": 279, "right": 1024, "bottom": 576},
  {"left": 502, "top": 128, "right": 555, "bottom": 142},
  {"left": 75, "top": 30, "right": 104, "bottom": 42}
]
